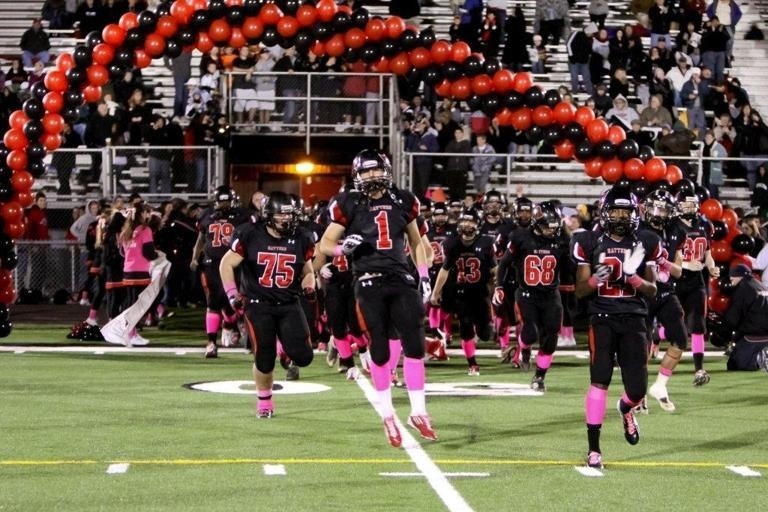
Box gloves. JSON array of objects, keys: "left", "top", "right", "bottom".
[
  {"left": 622, "top": 240, "right": 647, "bottom": 277},
  {"left": 492, "top": 286, "right": 505, "bottom": 306},
  {"left": 228, "top": 293, "right": 247, "bottom": 318},
  {"left": 588, "top": 262, "right": 613, "bottom": 289},
  {"left": 319, "top": 262, "right": 334, "bottom": 280},
  {"left": 341, "top": 234, "right": 364, "bottom": 257},
  {"left": 419, "top": 276, "right": 434, "bottom": 305}
]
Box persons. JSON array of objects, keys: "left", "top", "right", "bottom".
[
  {"left": 390, "top": 0, "right": 571, "bottom": 81},
  {"left": 0, "top": 0, "right": 132, "bottom": 137},
  {"left": 565, "top": 1, "right": 768, "bottom": 221},
  {"left": 51, "top": 44, "right": 562, "bottom": 202},
  {"left": 20, "top": 182, "right": 768, "bottom": 466}
]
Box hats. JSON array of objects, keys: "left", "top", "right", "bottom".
[{"left": 728, "top": 263, "right": 754, "bottom": 277}]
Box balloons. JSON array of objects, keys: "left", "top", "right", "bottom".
[
  {"left": 0, "top": 29, "right": 108, "bottom": 338},
  {"left": 100, "top": 2, "right": 474, "bottom": 97},
  {"left": 450, "top": 54, "right": 761, "bottom": 348}
]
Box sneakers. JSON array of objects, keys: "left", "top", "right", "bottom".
[
  {"left": 530, "top": 375, "right": 546, "bottom": 393},
  {"left": 79, "top": 296, "right": 173, "bottom": 344},
  {"left": 587, "top": 448, "right": 605, "bottom": 471},
  {"left": 650, "top": 385, "right": 676, "bottom": 413},
  {"left": 326, "top": 335, "right": 517, "bottom": 388},
  {"left": 650, "top": 326, "right": 660, "bottom": 361},
  {"left": 518, "top": 346, "right": 535, "bottom": 371},
  {"left": 759, "top": 346, "right": 768, "bottom": 373},
  {"left": 256, "top": 386, "right": 275, "bottom": 420},
  {"left": 406, "top": 411, "right": 438, "bottom": 442},
  {"left": 200, "top": 324, "right": 251, "bottom": 357},
  {"left": 556, "top": 336, "right": 577, "bottom": 347},
  {"left": 276, "top": 339, "right": 299, "bottom": 379},
  {"left": 616, "top": 399, "right": 641, "bottom": 445},
  {"left": 691, "top": 369, "right": 711, "bottom": 388},
  {"left": 383, "top": 413, "right": 402, "bottom": 449}
]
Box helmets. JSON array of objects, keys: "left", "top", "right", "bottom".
[
  {"left": 528, "top": 201, "right": 561, "bottom": 239},
  {"left": 259, "top": 190, "right": 301, "bottom": 240},
  {"left": 213, "top": 186, "right": 236, "bottom": 203},
  {"left": 432, "top": 200, "right": 450, "bottom": 228},
  {"left": 483, "top": 189, "right": 508, "bottom": 220},
  {"left": 644, "top": 190, "right": 676, "bottom": 229},
  {"left": 349, "top": 148, "right": 393, "bottom": 197},
  {"left": 457, "top": 210, "right": 482, "bottom": 237},
  {"left": 598, "top": 188, "right": 641, "bottom": 237},
  {"left": 512, "top": 196, "right": 533, "bottom": 226},
  {"left": 678, "top": 191, "right": 699, "bottom": 220}
]
